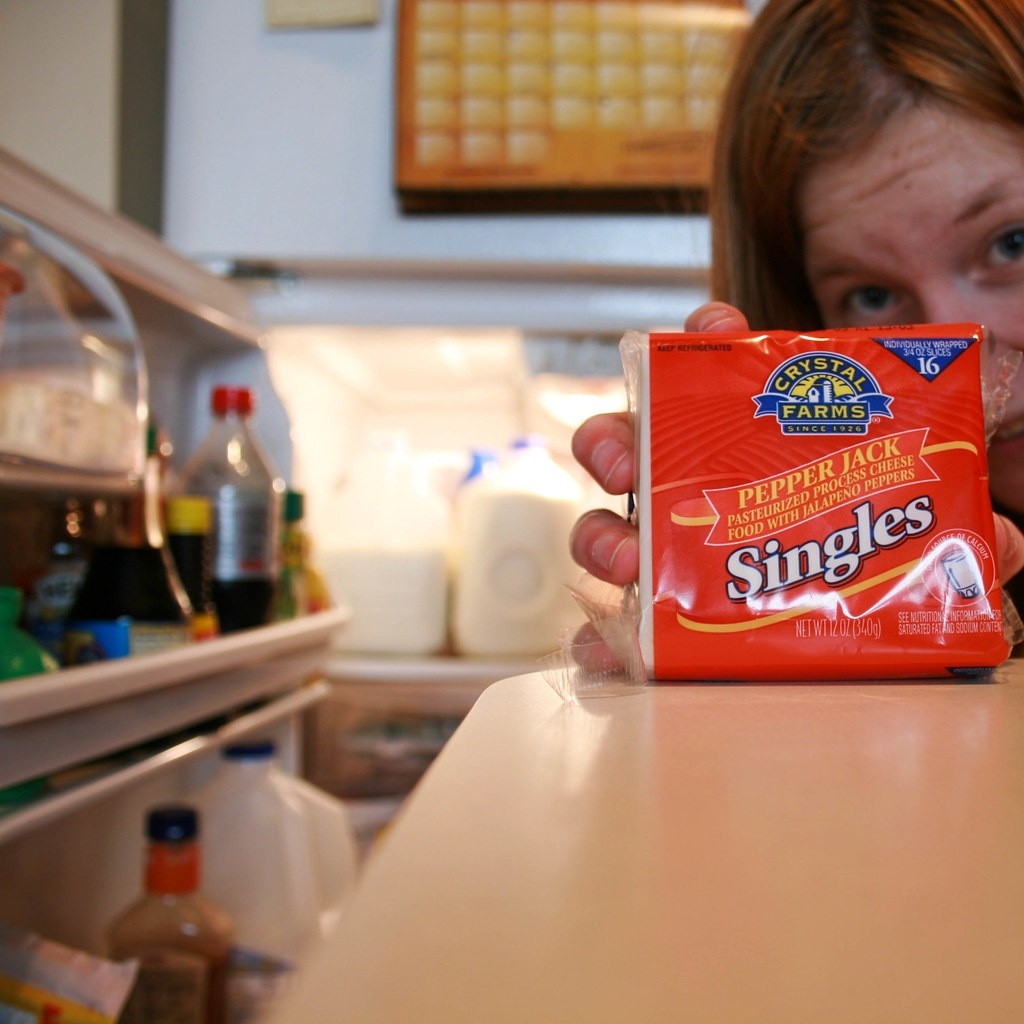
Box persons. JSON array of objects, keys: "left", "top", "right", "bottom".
[{"left": 571, "top": 0, "right": 1023, "bottom": 678}]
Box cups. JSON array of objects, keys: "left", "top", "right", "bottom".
[{"left": 76, "top": 622, "right": 132, "bottom": 664}]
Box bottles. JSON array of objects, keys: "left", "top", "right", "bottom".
[
  {"left": 186, "top": 740, "right": 357, "bottom": 1024},
  {"left": 102, "top": 802, "right": 238, "bottom": 1024},
  {"left": 0, "top": 586, "right": 52, "bottom": 808},
  {"left": 185, "top": 386, "right": 278, "bottom": 642},
  {"left": 280, "top": 492, "right": 310, "bottom": 618}
]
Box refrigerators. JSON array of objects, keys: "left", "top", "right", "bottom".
[{"left": 0, "top": 2, "right": 770, "bottom": 1023}]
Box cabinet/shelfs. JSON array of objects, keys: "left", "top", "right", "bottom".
[{"left": 0, "top": 156, "right": 344, "bottom": 1024}]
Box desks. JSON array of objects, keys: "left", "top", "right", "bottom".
[{"left": 281, "top": 657, "right": 1024, "bottom": 1024}]
